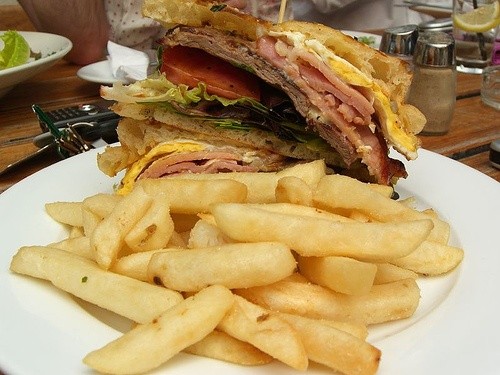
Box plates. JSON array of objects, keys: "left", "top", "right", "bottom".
[
  {"left": 1, "top": 140, "right": 499, "bottom": 375},
  {"left": 77, "top": 60, "right": 135, "bottom": 87}
]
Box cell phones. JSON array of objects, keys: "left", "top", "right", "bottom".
[{"left": 39, "top": 100, "right": 117, "bottom": 133}]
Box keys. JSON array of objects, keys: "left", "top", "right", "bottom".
[{"left": 32, "top": 103, "right": 95, "bottom": 159}]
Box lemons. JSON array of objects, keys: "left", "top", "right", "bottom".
[{"left": 452, "top": 1, "right": 499, "bottom": 33}]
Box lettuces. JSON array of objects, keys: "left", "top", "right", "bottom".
[{"left": 0, "top": 30, "right": 30, "bottom": 70}]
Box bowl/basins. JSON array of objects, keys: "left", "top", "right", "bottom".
[{"left": 1, "top": 27, "right": 73, "bottom": 97}]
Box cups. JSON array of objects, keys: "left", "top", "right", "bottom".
[{"left": 451, "top": 0, "right": 500, "bottom": 76}]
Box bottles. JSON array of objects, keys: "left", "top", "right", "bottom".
[
  {"left": 404, "top": 29, "right": 458, "bottom": 137},
  {"left": 378, "top": 22, "right": 420, "bottom": 134}
]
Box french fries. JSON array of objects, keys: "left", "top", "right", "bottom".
[{"left": 11, "top": 156, "right": 465, "bottom": 375}]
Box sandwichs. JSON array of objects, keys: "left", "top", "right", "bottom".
[{"left": 100, "top": 1, "right": 426, "bottom": 196}]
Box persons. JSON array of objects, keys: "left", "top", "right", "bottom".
[{"left": 16, "top": 0, "right": 110, "bottom": 67}]
